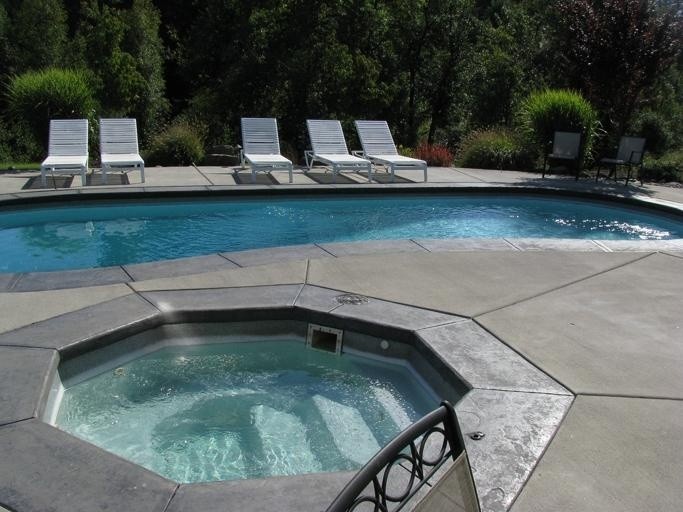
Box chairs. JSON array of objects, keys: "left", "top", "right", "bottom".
[
  {"left": 39, "top": 118, "right": 146, "bottom": 185},
  {"left": 236, "top": 116, "right": 428, "bottom": 183},
  {"left": 541, "top": 131, "right": 647, "bottom": 187}
]
